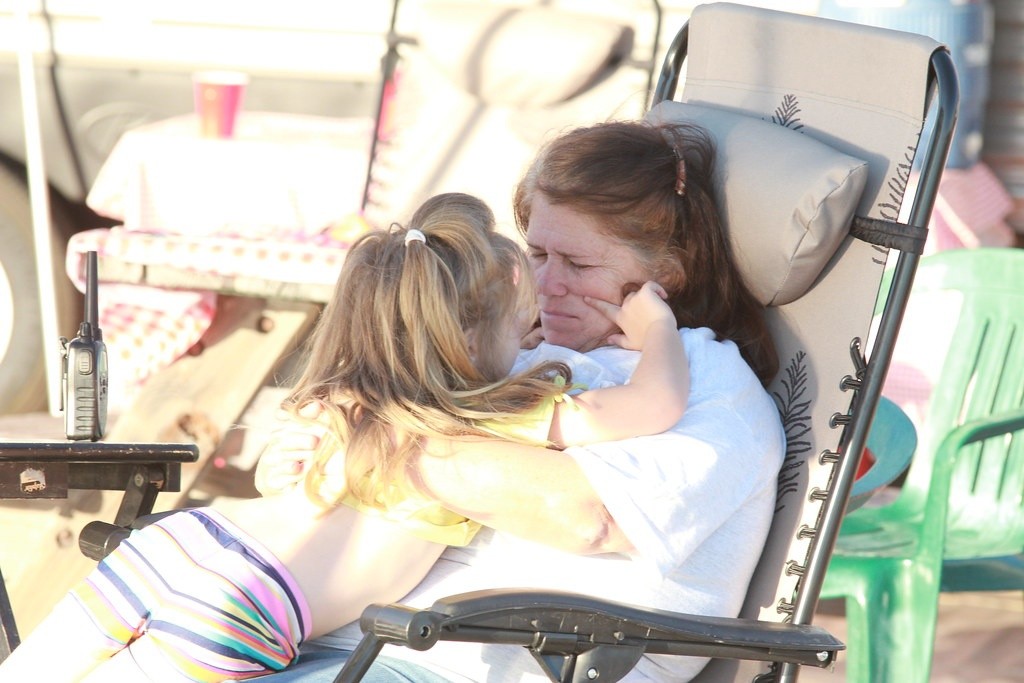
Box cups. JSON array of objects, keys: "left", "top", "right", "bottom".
[{"left": 190, "top": 71, "right": 250, "bottom": 141}]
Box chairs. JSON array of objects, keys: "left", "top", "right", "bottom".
[
  {"left": 80, "top": 4, "right": 956, "bottom": 683},
  {"left": 818, "top": 246, "right": 1024, "bottom": 683}
]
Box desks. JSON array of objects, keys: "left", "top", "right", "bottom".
[
  {"left": 86, "top": 109, "right": 375, "bottom": 231},
  {"left": 0, "top": 442, "right": 199, "bottom": 672}
]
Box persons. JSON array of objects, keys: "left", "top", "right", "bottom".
[
  {"left": 249, "top": 122, "right": 789, "bottom": 682},
  {"left": 0, "top": 191, "right": 691, "bottom": 681}
]
